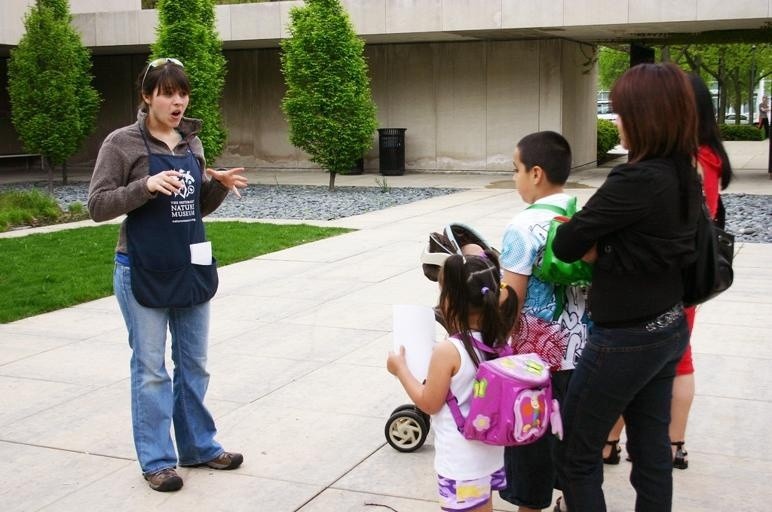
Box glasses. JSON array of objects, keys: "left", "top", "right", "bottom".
[{"left": 142, "top": 58, "right": 184, "bottom": 88}]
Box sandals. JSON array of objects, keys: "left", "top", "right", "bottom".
[
  {"left": 602, "top": 440, "right": 621, "bottom": 464},
  {"left": 672, "top": 442, "right": 688, "bottom": 469}
]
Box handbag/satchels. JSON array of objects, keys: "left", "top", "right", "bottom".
[{"left": 683, "top": 203, "right": 732, "bottom": 308}]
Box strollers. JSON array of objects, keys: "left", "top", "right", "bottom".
[{"left": 383, "top": 223, "right": 500, "bottom": 452}]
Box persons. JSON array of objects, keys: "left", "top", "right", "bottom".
[
  {"left": 85, "top": 55, "right": 248, "bottom": 495},
  {"left": 757, "top": 95, "right": 770, "bottom": 138},
  {"left": 550, "top": 62, "right": 734, "bottom": 512},
  {"left": 498, "top": 131, "right": 590, "bottom": 512},
  {"left": 387, "top": 223, "right": 519, "bottom": 511}
]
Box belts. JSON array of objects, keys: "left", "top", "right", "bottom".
[{"left": 646, "top": 302, "right": 684, "bottom": 335}]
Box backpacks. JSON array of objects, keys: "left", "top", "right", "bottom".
[
  {"left": 525, "top": 197, "right": 593, "bottom": 289},
  {"left": 445, "top": 333, "right": 553, "bottom": 446}
]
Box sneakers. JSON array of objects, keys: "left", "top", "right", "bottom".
[
  {"left": 208, "top": 452, "right": 243, "bottom": 470},
  {"left": 144, "top": 469, "right": 182, "bottom": 492}
]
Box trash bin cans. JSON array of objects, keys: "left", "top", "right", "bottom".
[
  {"left": 377, "top": 127, "right": 407, "bottom": 175},
  {"left": 341, "top": 140, "right": 364, "bottom": 175}
]
